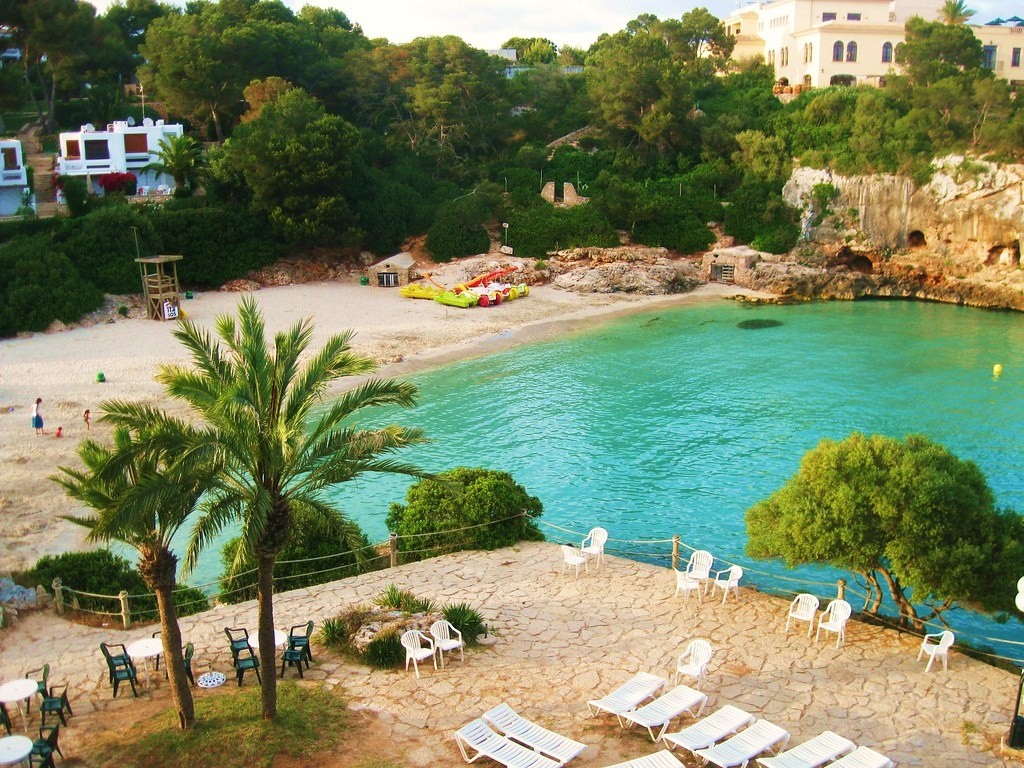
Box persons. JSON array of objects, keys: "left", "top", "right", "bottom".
[
  {"left": 55, "top": 427, "right": 62, "bottom": 438},
  {"left": 83, "top": 410, "right": 91, "bottom": 430},
  {"left": 31, "top": 397, "right": 46, "bottom": 436}
]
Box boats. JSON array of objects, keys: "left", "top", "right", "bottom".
[{"left": 400, "top": 267, "right": 529, "bottom": 308}]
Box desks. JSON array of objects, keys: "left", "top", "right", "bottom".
[
  {"left": 0, "top": 735, "right": 33, "bottom": 768},
  {"left": 248, "top": 629, "right": 288, "bottom": 669},
  {"left": 0, "top": 679, "right": 43, "bottom": 733},
  {"left": 126, "top": 638, "right": 164, "bottom": 688}
]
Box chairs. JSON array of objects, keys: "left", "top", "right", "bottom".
[
  {"left": 916, "top": 630, "right": 955, "bottom": 672},
  {"left": 561, "top": 544, "right": 588, "bottom": 581},
  {"left": 224, "top": 621, "right": 314, "bottom": 687},
  {"left": 587, "top": 671, "right": 667, "bottom": 730},
  {"left": 400, "top": 630, "right": 438, "bottom": 679},
  {"left": 580, "top": 528, "right": 608, "bottom": 569},
  {"left": 673, "top": 567, "right": 702, "bottom": 604},
  {"left": 711, "top": 565, "right": 743, "bottom": 605},
  {"left": 0, "top": 664, "right": 73, "bottom": 768},
  {"left": 675, "top": 640, "right": 712, "bottom": 692},
  {"left": 601, "top": 749, "right": 686, "bottom": 768},
  {"left": 755, "top": 731, "right": 858, "bottom": 768},
  {"left": 693, "top": 719, "right": 790, "bottom": 768},
  {"left": 823, "top": 746, "right": 893, "bottom": 768},
  {"left": 455, "top": 718, "right": 563, "bottom": 768},
  {"left": 430, "top": 620, "right": 464, "bottom": 668},
  {"left": 482, "top": 703, "right": 588, "bottom": 766},
  {"left": 620, "top": 685, "right": 708, "bottom": 744},
  {"left": 686, "top": 550, "right": 714, "bottom": 595},
  {"left": 816, "top": 599, "right": 852, "bottom": 649},
  {"left": 100, "top": 630, "right": 195, "bottom": 698},
  {"left": 786, "top": 594, "right": 819, "bottom": 638},
  {"left": 662, "top": 705, "right": 754, "bottom": 765}
]
